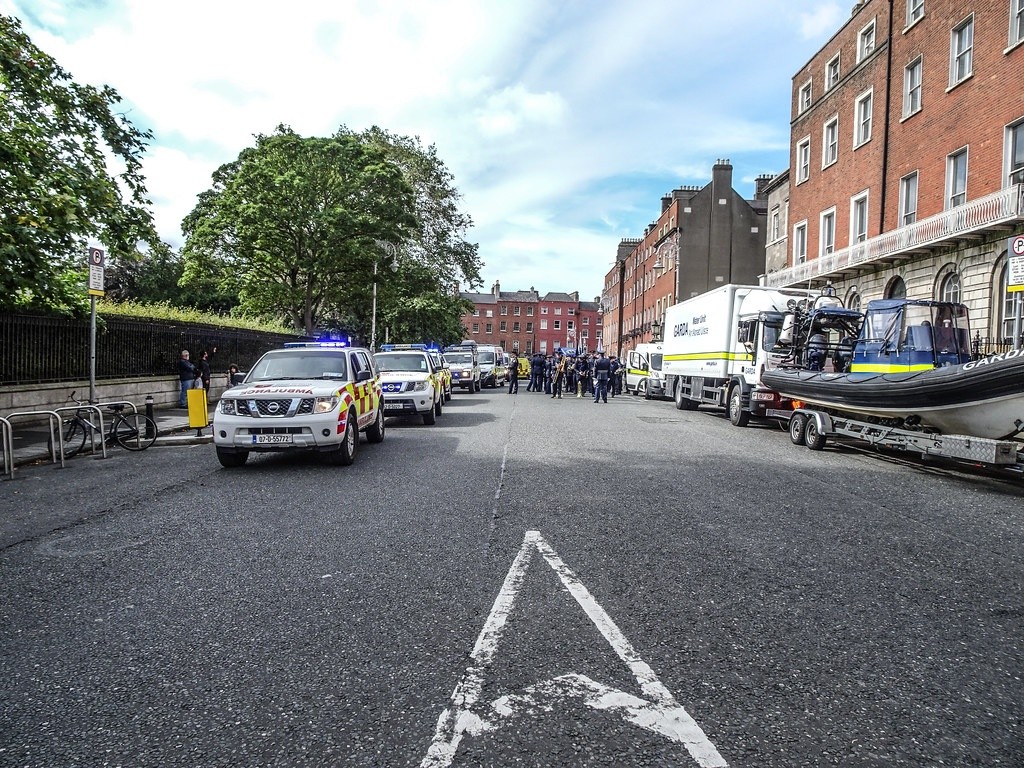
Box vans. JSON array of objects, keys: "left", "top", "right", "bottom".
[
  {"left": 477, "top": 344, "right": 514, "bottom": 388},
  {"left": 626, "top": 342, "right": 666, "bottom": 399},
  {"left": 515, "top": 358, "right": 530, "bottom": 379}
]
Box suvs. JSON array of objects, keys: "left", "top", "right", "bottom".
[
  {"left": 368, "top": 343, "right": 443, "bottom": 424},
  {"left": 444, "top": 347, "right": 481, "bottom": 393},
  {"left": 211, "top": 341, "right": 386, "bottom": 468},
  {"left": 427, "top": 349, "right": 453, "bottom": 404}
]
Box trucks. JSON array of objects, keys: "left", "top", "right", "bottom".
[{"left": 554, "top": 347, "right": 577, "bottom": 361}]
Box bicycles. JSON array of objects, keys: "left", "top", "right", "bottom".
[{"left": 48, "top": 390, "right": 158, "bottom": 460}]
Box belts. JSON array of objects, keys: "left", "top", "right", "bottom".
[{"left": 597, "top": 370, "right": 608, "bottom": 372}]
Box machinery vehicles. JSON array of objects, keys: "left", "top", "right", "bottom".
[{"left": 777, "top": 286, "right": 994, "bottom": 379}]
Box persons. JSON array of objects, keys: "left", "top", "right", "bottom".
[
  {"left": 526, "top": 347, "right": 623, "bottom": 403},
  {"left": 179, "top": 350, "right": 196, "bottom": 409},
  {"left": 226, "top": 364, "right": 244, "bottom": 389},
  {"left": 506, "top": 351, "right": 518, "bottom": 394},
  {"left": 199, "top": 351, "right": 212, "bottom": 404},
  {"left": 194, "top": 370, "right": 203, "bottom": 388}
]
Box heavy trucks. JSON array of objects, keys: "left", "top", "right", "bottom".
[{"left": 661, "top": 282, "right": 830, "bottom": 427}]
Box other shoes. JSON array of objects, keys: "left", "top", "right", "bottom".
[
  {"left": 604, "top": 399, "right": 607, "bottom": 403},
  {"left": 594, "top": 401, "right": 598, "bottom": 403},
  {"left": 513, "top": 391, "right": 517, "bottom": 394},
  {"left": 551, "top": 395, "right": 555, "bottom": 398},
  {"left": 180, "top": 406, "right": 188, "bottom": 408},
  {"left": 526, "top": 387, "right": 530, "bottom": 391},
  {"left": 558, "top": 395, "right": 562, "bottom": 399},
  {"left": 207, "top": 398, "right": 212, "bottom": 404}
]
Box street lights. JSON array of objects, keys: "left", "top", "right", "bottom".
[{"left": 372, "top": 241, "right": 400, "bottom": 354}]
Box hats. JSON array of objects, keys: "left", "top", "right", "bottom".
[
  {"left": 599, "top": 351, "right": 604, "bottom": 354},
  {"left": 577, "top": 355, "right": 584, "bottom": 357},
  {"left": 538, "top": 353, "right": 542, "bottom": 356},
  {"left": 590, "top": 350, "right": 595, "bottom": 353},
  {"left": 608, "top": 356, "right": 615, "bottom": 359}
]
